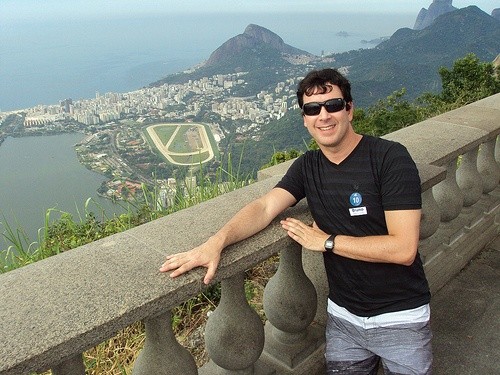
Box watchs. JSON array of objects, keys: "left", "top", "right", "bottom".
[{"left": 324, "top": 234, "right": 338, "bottom": 254}]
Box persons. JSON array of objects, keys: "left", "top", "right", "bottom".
[{"left": 160, "top": 68, "right": 434, "bottom": 375}]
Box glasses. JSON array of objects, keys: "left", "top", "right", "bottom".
[{"left": 302, "top": 98, "right": 347, "bottom": 116}]
